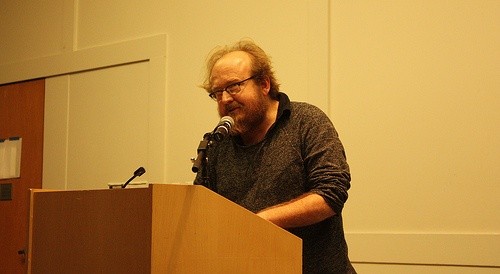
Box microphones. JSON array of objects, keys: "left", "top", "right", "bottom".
[{"left": 214, "top": 116, "right": 234, "bottom": 143}]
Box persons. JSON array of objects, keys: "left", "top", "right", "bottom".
[{"left": 190, "top": 40, "right": 357, "bottom": 274}]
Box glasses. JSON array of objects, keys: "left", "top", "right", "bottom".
[{"left": 209, "top": 73, "right": 257, "bottom": 102}]
